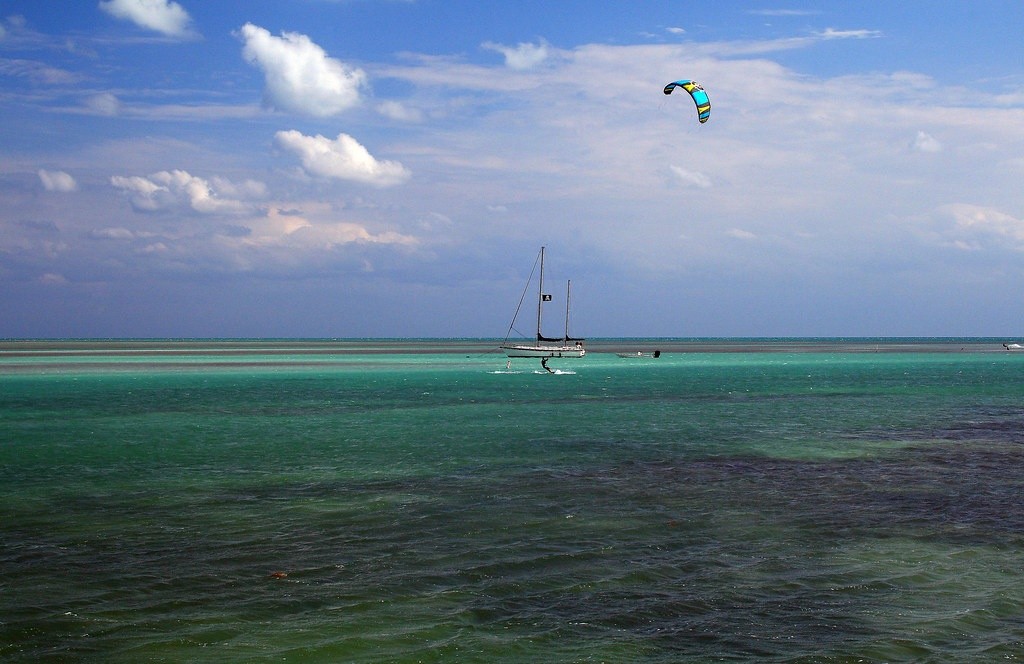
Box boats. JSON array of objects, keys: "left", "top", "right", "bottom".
[
  {"left": 499, "top": 246, "right": 586, "bottom": 359},
  {"left": 615, "top": 350, "right": 661, "bottom": 359}
]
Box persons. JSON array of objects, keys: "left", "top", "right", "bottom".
[{"left": 541, "top": 356, "right": 555, "bottom": 373}]
